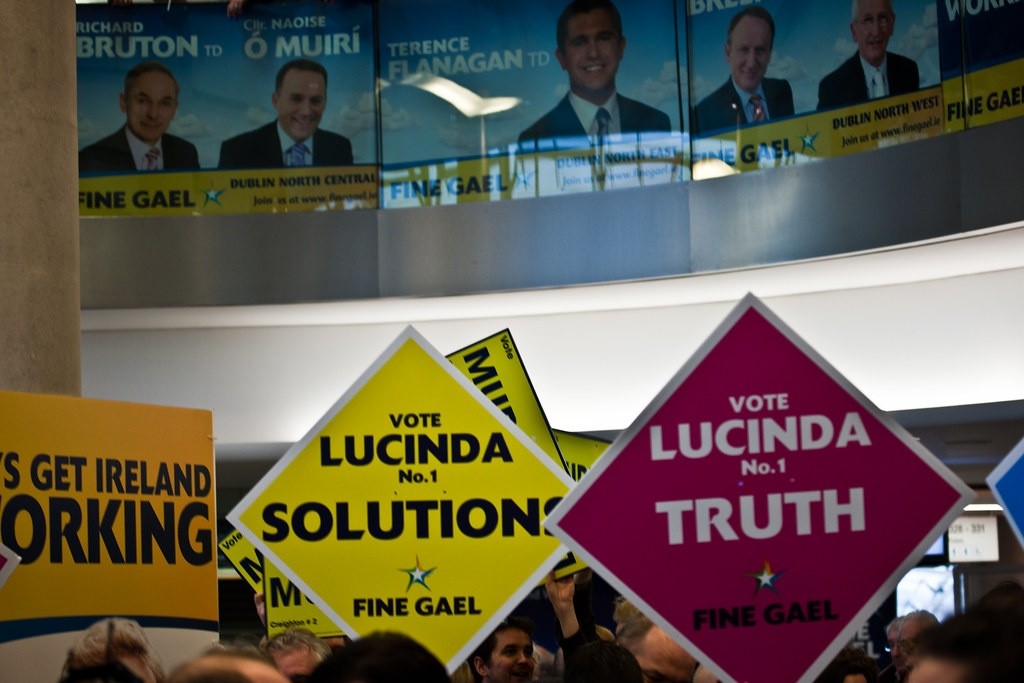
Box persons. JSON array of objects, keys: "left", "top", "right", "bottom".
[
  {"left": 219, "top": 61, "right": 354, "bottom": 169},
  {"left": 816, "top": 1, "right": 920, "bottom": 111},
  {"left": 58, "top": 566, "right": 1024, "bottom": 683},
  {"left": 692, "top": 6, "right": 796, "bottom": 138},
  {"left": 78, "top": 62, "right": 200, "bottom": 178},
  {"left": 517, "top": 1, "right": 671, "bottom": 143}
]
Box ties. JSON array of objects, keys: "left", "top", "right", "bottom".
[
  {"left": 594, "top": 107, "right": 612, "bottom": 147},
  {"left": 289, "top": 144, "right": 307, "bottom": 166},
  {"left": 873, "top": 71, "right": 885, "bottom": 97},
  {"left": 145, "top": 148, "right": 160, "bottom": 170},
  {"left": 749, "top": 96, "right": 768, "bottom": 123}
]
